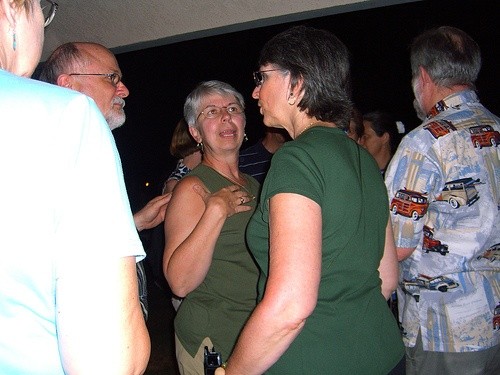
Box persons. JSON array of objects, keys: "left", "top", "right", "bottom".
[
  {"left": 161, "top": 79, "right": 265, "bottom": 375},
  {"left": 357, "top": 112, "right": 398, "bottom": 179},
  {"left": 2, "top": 1, "right": 155, "bottom": 374},
  {"left": 37, "top": 40, "right": 174, "bottom": 327},
  {"left": 213, "top": 23, "right": 402, "bottom": 375},
  {"left": 161, "top": 123, "right": 204, "bottom": 197},
  {"left": 340, "top": 108, "right": 366, "bottom": 145},
  {"left": 238, "top": 125, "right": 293, "bottom": 187},
  {"left": 389, "top": 24, "right": 500, "bottom": 374}
]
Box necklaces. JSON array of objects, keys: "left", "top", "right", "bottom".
[
  {"left": 212, "top": 166, "right": 251, "bottom": 194},
  {"left": 296, "top": 122, "right": 313, "bottom": 137}
]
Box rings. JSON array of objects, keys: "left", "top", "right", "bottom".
[{"left": 239, "top": 197, "right": 244, "bottom": 205}]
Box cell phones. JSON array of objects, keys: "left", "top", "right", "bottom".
[{"left": 203, "top": 346, "right": 222, "bottom": 375}]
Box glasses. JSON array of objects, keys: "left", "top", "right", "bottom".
[
  {"left": 253, "top": 69, "right": 291, "bottom": 87},
  {"left": 345, "top": 127, "right": 356, "bottom": 136},
  {"left": 195, "top": 102, "right": 245, "bottom": 125},
  {"left": 69, "top": 73, "right": 122, "bottom": 86},
  {"left": 40, "top": 0, "right": 59, "bottom": 28}
]
